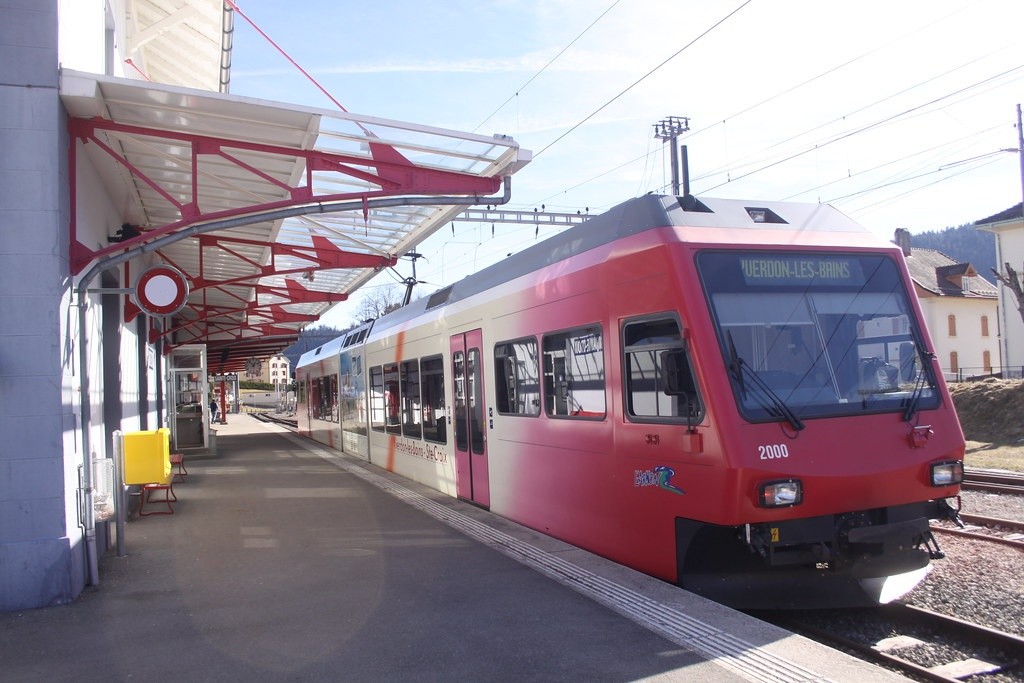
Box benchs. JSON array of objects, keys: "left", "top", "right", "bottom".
[
  {"left": 137, "top": 472, "right": 176, "bottom": 516},
  {"left": 171, "top": 453, "right": 187, "bottom": 483}
]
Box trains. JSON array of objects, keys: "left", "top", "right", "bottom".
[{"left": 290, "top": 190, "right": 968, "bottom": 594}]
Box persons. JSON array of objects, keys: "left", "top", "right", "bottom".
[{"left": 210, "top": 398, "right": 218, "bottom": 424}]
